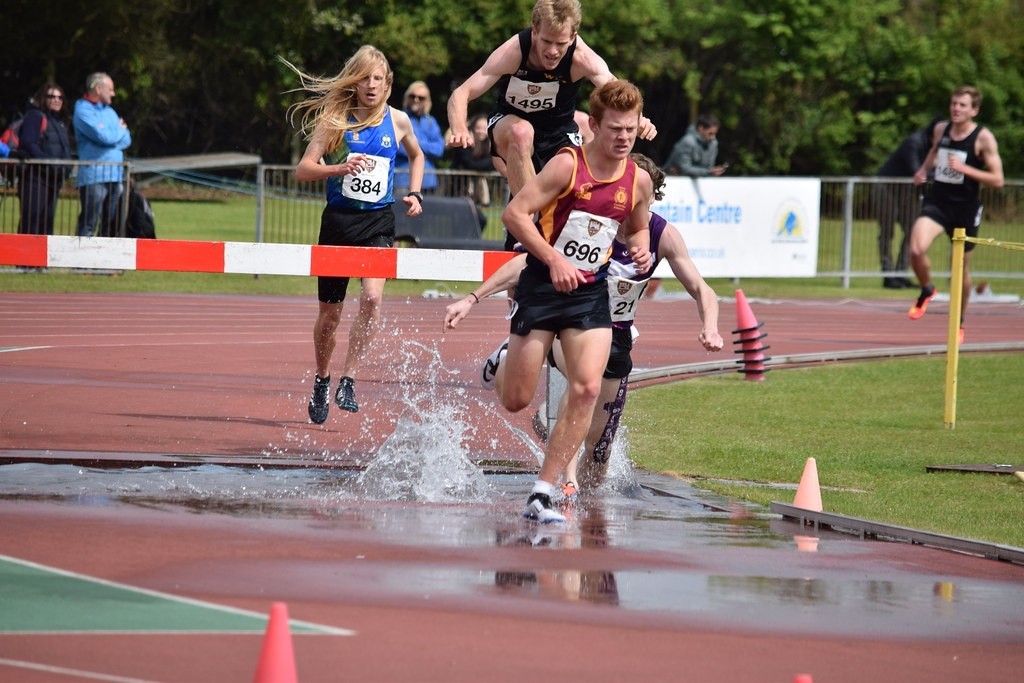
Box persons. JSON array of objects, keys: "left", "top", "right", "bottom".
[
  {"left": 283, "top": 44, "right": 425, "bottom": 426},
  {"left": 482, "top": 79, "right": 653, "bottom": 522},
  {"left": 662, "top": 113, "right": 727, "bottom": 176},
  {"left": 440, "top": 154, "right": 723, "bottom": 485},
  {"left": 907, "top": 85, "right": 1003, "bottom": 348},
  {"left": 394, "top": 80, "right": 446, "bottom": 193},
  {"left": 73, "top": 72, "right": 132, "bottom": 236},
  {"left": 451, "top": 115, "right": 490, "bottom": 205},
  {"left": 18, "top": 76, "right": 72, "bottom": 272},
  {"left": 446, "top": 2, "right": 657, "bottom": 251},
  {"left": 866, "top": 117, "right": 939, "bottom": 288}
]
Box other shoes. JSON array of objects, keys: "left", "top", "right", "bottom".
[{"left": 884, "top": 277, "right": 913, "bottom": 288}]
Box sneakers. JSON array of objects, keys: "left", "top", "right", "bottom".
[
  {"left": 334, "top": 378, "right": 358, "bottom": 413},
  {"left": 308, "top": 382, "right": 329, "bottom": 425},
  {"left": 522, "top": 493, "right": 566, "bottom": 523},
  {"left": 908, "top": 285, "right": 935, "bottom": 320},
  {"left": 960, "top": 325, "right": 964, "bottom": 343},
  {"left": 480, "top": 336, "right": 509, "bottom": 390}
]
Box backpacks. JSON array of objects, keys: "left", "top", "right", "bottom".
[{"left": 109, "top": 186, "right": 156, "bottom": 239}]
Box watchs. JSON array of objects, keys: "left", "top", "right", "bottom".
[{"left": 407, "top": 191, "right": 424, "bottom": 203}]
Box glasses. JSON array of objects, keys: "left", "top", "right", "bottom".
[
  {"left": 45, "top": 95, "right": 63, "bottom": 100},
  {"left": 408, "top": 94, "right": 425, "bottom": 101}
]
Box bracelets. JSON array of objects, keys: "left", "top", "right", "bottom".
[{"left": 469, "top": 293, "right": 480, "bottom": 303}]
